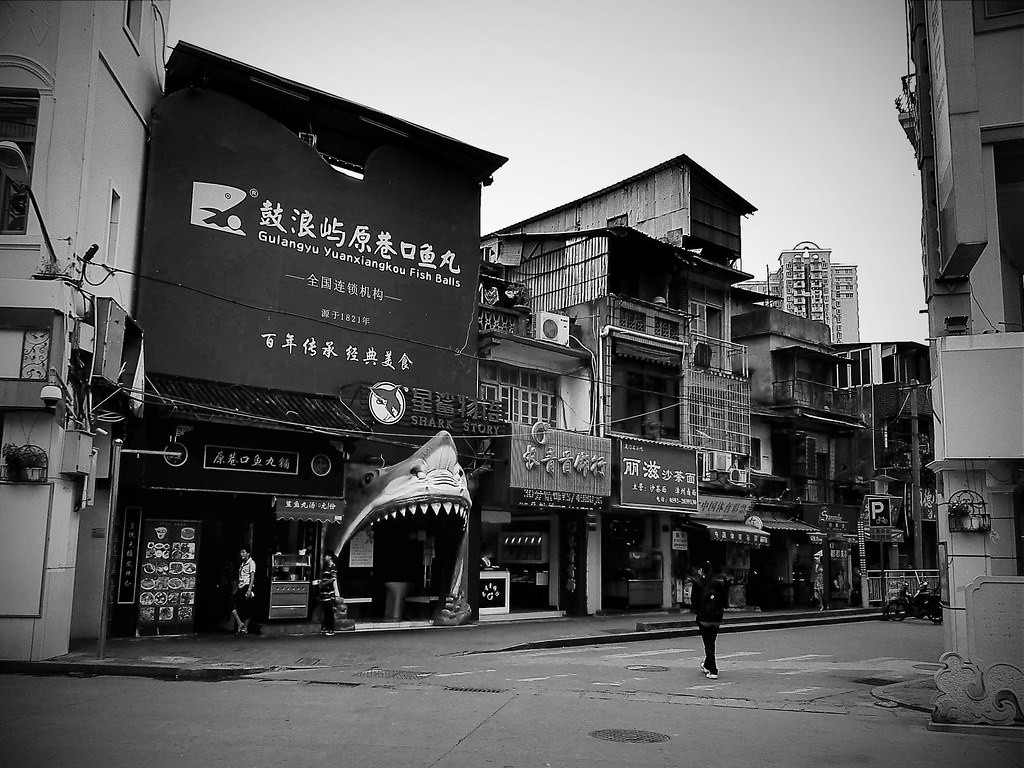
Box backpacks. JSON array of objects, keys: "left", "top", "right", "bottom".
[{"left": 695, "top": 578, "right": 723, "bottom": 629}]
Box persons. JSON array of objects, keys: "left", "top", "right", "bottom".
[
  {"left": 316, "top": 549, "right": 338, "bottom": 636},
  {"left": 815, "top": 564, "right": 919, "bottom": 611},
  {"left": 232, "top": 549, "right": 256, "bottom": 636},
  {"left": 691, "top": 561, "right": 726, "bottom": 679}
]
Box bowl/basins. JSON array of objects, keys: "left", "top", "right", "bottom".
[{"left": 146, "top": 542, "right": 170, "bottom": 559}]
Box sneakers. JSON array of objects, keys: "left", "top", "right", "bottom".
[
  {"left": 700, "top": 661, "right": 710, "bottom": 673},
  {"left": 705, "top": 671, "right": 718, "bottom": 679}
]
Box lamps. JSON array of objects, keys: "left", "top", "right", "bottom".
[{"left": 40, "top": 367, "right": 62, "bottom": 406}]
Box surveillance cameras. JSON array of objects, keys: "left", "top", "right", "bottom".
[{"left": 39, "top": 384, "right": 63, "bottom": 406}]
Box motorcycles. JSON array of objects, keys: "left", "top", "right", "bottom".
[{"left": 886, "top": 576, "right": 943, "bottom": 625}]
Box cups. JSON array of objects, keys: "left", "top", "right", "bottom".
[{"left": 156, "top": 527, "right": 167, "bottom": 539}]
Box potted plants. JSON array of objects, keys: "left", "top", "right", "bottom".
[
  {"left": 2, "top": 443, "right": 48, "bottom": 482},
  {"left": 948, "top": 490, "right": 991, "bottom": 533}
]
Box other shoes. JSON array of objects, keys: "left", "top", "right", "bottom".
[
  {"left": 321, "top": 629, "right": 329, "bottom": 633},
  {"left": 235, "top": 622, "right": 244, "bottom": 635},
  {"left": 817, "top": 607, "right": 821, "bottom": 611},
  {"left": 325, "top": 632, "right": 335, "bottom": 636},
  {"left": 820, "top": 607, "right": 825, "bottom": 611},
  {"left": 241, "top": 628, "right": 248, "bottom": 634}
]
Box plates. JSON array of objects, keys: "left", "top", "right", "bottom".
[
  {"left": 169, "top": 562, "right": 183, "bottom": 574},
  {"left": 154, "top": 592, "right": 167, "bottom": 605},
  {"left": 167, "top": 577, "right": 183, "bottom": 590},
  {"left": 183, "top": 563, "right": 196, "bottom": 574},
  {"left": 141, "top": 578, "right": 155, "bottom": 590},
  {"left": 144, "top": 563, "right": 155, "bottom": 573},
  {"left": 159, "top": 607, "right": 173, "bottom": 620},
  {"left": 181, "top": 527, "right": 195, "bottom": 540},
  {"left": 172, "top": 550, "right": 183, "bottom": 559},
  {"left": 139, "top": 592, "right": 154, "bottom": 606}
]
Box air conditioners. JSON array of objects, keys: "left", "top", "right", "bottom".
[
  {"left": 709, "top": 451, "right": 732, "bottom": 472},
  {"left": 532, "top": 311, "right": 570, "bottom": 348},
  {"left": 729, "top": 468, "right": 747, "bottom": 487}
]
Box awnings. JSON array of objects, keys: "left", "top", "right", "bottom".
[
  {"left": 750, "top": 511, "right": 820, "bottom": 532},
  {"left": 687, "top": 519, "right": 770, "bottom": 546}
]
